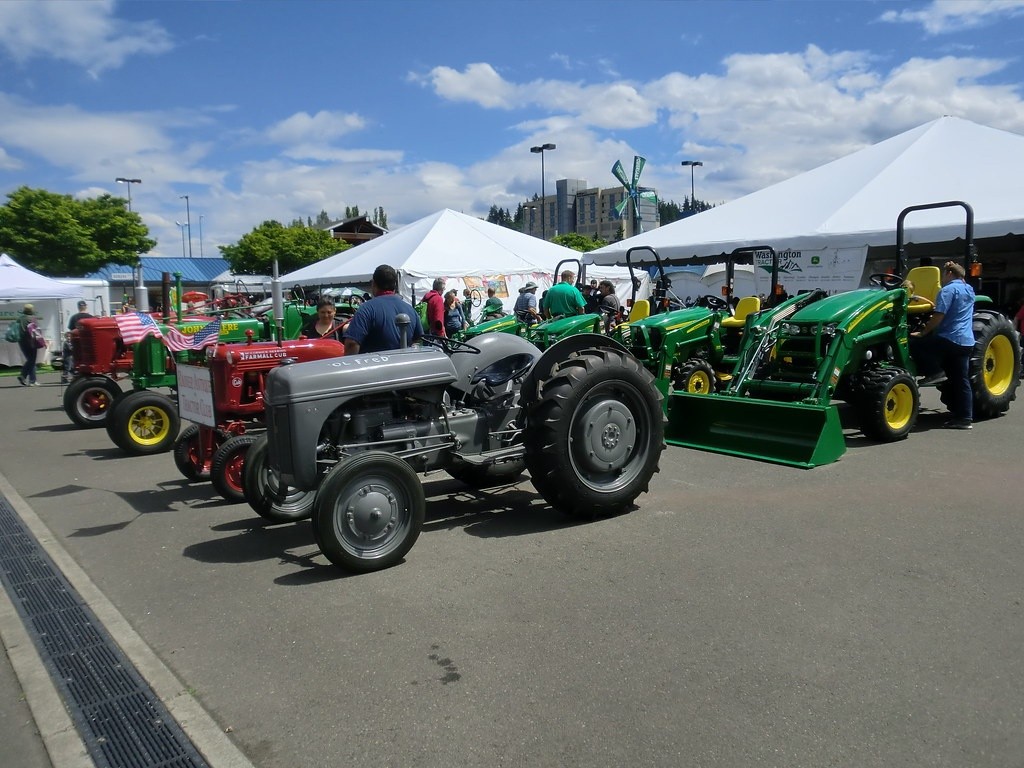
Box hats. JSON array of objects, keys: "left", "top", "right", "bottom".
[
  {"left": 524, "top": 282, "right": 539, "bottom": 290},
  {"left": 24, "top": 304, "right": 34, "bottom": 313},
  {"left": 77, "top": 300, "right": 87, "bottom": 307}
]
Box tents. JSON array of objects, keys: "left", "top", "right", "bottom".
[
  {"left": 263, "top": 208, "right": 649, "bottom": 325},
  {"left": 0, "top": 253, "right": 85, "bottom": 300},
  {"left": 583, "top": 115, "right": 1024, "bottom": 287}
]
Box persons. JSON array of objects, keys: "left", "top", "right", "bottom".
[
  {"left": 515, "top": 282, "right": 542, "bottom": 325},
  {"left": 423, "top": 280, "right": 447, "bottom": 338},
  {"left": 444, "top": 289, "right": 472, "bottom": 339},
  {"left": 583, "top": 280, "right": 600, "bottom": 314},
  {"left": 62, "top": 332, "right": 73, "bottom": 383},
  {"left": 599, "top": 281, "right": 620, "bottom": 325},
  {"left": 17, "top": 304, "right": 41, "bottom": 386},
  {"left": 486, "top": 287, "right": 502, "bottom": 314},
  {"left": 910, "top": 262, "right": 976, "bottom": 429},
  {"left": 302, "top": 297, "right": 349, "bottom": 343},
  {"left": 343, "top": 265, "right": 424, "bottom": 356},
  {"left": 543, "top": 270, "right": 587, "bottom": 319},
  {"left": 68, "top": 301, "right": 93, "bottom": 330},
  {"left": 539, "top": 290, "right": 548, "bottom": 321}
]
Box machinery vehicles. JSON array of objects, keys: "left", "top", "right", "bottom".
[
  {"left": 239, "top": 312, "right": 670, "bottom": 576},
  {"left": 50, "top": 260, "right": 832, "bottom": 504},
  {"left": 662, "top": 199, "right": 1024, "bottom": 469}
]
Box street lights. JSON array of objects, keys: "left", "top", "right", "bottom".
[
  {"left": 176, "top": 221, "right": 189, "bottom": 257},
  {"left": 199, "top": 215, "right": 203, "bottom": 258},
  {"left": 530, "top": 143, "right": 557, "bottom": 240},
  {"left": 682, "top": 161, "right": 703, "bottom": 214},
  {"left": 115, "top": 178, "right": 142, "bottom": 211},
  {"left": 180, "top": 195, "right": 192, "bottom": 257}
]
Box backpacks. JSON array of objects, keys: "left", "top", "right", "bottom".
[{"left": 412, "top": 300, "right": 430, "bottom": 332}]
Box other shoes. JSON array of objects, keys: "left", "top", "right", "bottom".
[
  {"left": 29, "top": 382, "right": 40, "bottom": 386},
  {"left": 61, "top": 379, "right": 70, "bottom": 384},
  {"left": 18, "top": 375, "right": 27, "bottom": 385}
]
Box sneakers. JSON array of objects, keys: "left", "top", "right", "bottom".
[
  {"left": 940, "top": 417, "right": 973, "bottom": 429},
  {"left": 917, "top": 371, "right": 948, "bottom": 385}
]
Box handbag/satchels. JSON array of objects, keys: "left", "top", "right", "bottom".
[
  {"left": 3, "top": 322, "right": 20, "bottom": 343},
  {"left": 27, "top": 316, "right": 45, "bottom": 349}
]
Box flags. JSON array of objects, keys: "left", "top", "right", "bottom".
[
  {"left": 163, "top": 318, "right": 221, "bottom": 351},
  {"left": 114, "top": 312, "right": 162, "bottom": 344}
]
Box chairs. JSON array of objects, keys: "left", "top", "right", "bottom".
[
  {"left": 720, "top": 298, "right": 761, "bottom": 328},
  {"left": 901, "top": 266, "right": 942, "bottom": 313},
  {"left": 615, "top": 300, "right": 650, "bottom": 329}
]
